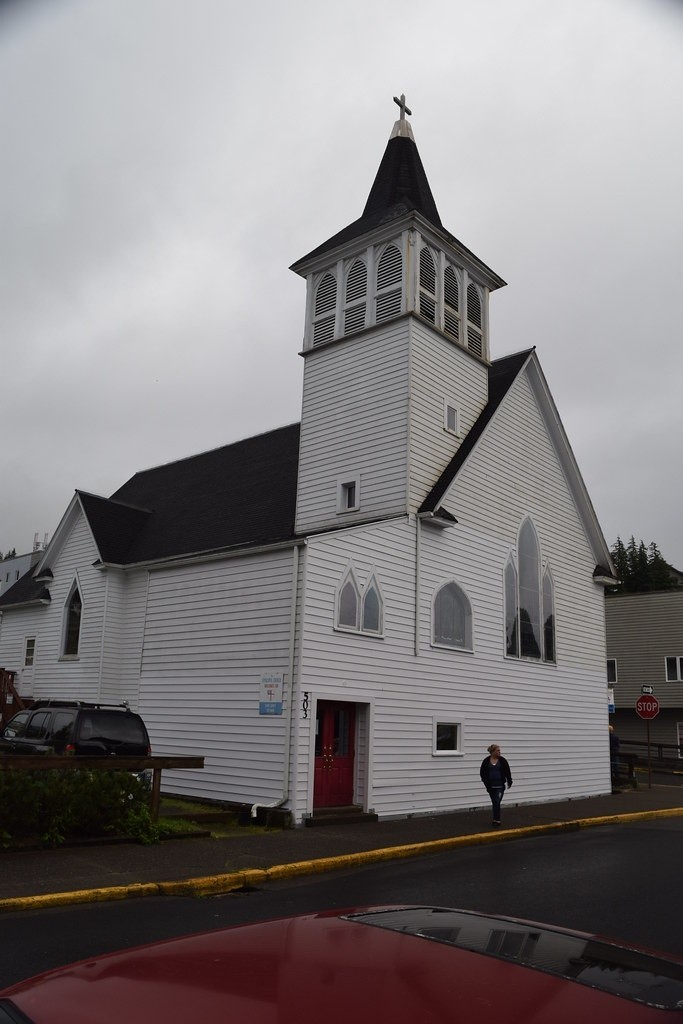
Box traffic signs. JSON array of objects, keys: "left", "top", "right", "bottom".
[{"left": 642, "top": 684, "right": 654, "bottom": 695}]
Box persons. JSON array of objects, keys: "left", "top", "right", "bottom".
[
  {"left": 479, "top": 744, "right": 513, "bottom": 826},
  {"left": 609, "top": 726, "right": 620, "bottom": 779}
]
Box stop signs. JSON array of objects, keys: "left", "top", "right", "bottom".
[{"left": 635, "top": 695, "right": 659, "bottom": 719}]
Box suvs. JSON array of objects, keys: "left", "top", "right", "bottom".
[{"left": 0, "top": 695, "right": 152, "bottom": 789}]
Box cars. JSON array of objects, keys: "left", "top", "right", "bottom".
[{"left": 0, "top": 902, "right": 682, "bottom": 1024}]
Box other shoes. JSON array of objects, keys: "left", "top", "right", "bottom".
[{"left": 492, "top": 820, "right": 501, "bottom": 826}]
